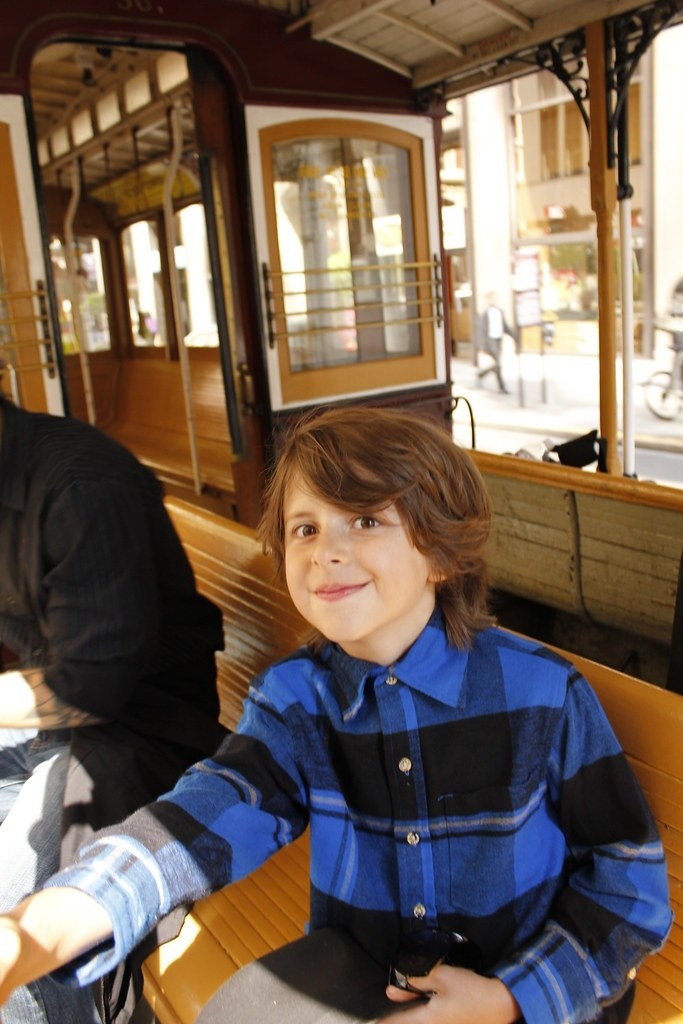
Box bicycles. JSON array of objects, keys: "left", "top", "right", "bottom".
[{"left": 640, "top": 335, "right": 683, "bottom": 421}]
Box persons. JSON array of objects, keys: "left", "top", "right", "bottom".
[
  {"left": 476, "top": 295, "right": 518, "bottom": 392},
  {"left": 0, "top": 369, "right": 219, "bottom": 1023},
  {"left": 0, "top": 401, "right": 676, "bottom": 1024}
]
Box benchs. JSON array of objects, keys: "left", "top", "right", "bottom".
[{"left": 138, "top": 446, "right": 683, "bottom": 1024}]
